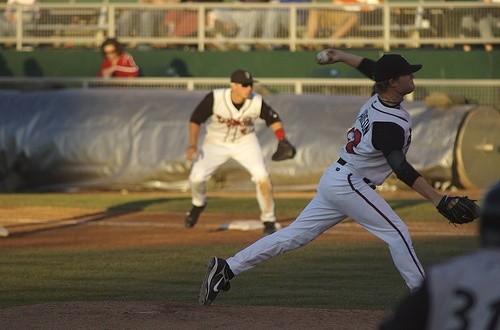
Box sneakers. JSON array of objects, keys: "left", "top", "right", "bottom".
[
  {"left": 263, "top": 222, "right": 276, "bottom": 235},
  {"left": 185, "top": 202, "right": 207, "bottom": 228},
  {"left": 198, "top": 256, "right": 231, "bottom": 308}
]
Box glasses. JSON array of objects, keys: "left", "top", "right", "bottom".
[
  {"left": 242, "top": 83, "right": 253, "bottom": 87},
  {"left": 104, "top": 48, "right": 117, "bottom": 55}
]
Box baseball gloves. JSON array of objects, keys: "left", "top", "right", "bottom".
[
  {"left": 272, "top": 140, "right": 296, "bottom": 162},
  {"left": 438, "top": 195, "right": 480, "bottom": 227}
]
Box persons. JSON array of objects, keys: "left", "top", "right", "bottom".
[
  {"left": 199, "top": 49, "right": 482, "bottom": 306},
  {"left": 378, "top": 181, "right": 500, "bottom": 330},
  {"left": 98, "top": 38, "right": 140, "bottom": 77},
  {"left": 186, "top": 70, "right": 294, "bottom": 235}
]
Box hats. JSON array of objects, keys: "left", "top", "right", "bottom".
[
  {"left": 230, "top": 69, "right": 258, "bottom": 85},
  {"left": 372, "top": 53, "right": 423, "bottom": 81}
]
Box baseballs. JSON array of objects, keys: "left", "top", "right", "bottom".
[{"left": 316, "top": 50, "right": 329, "bottom": 64}]
